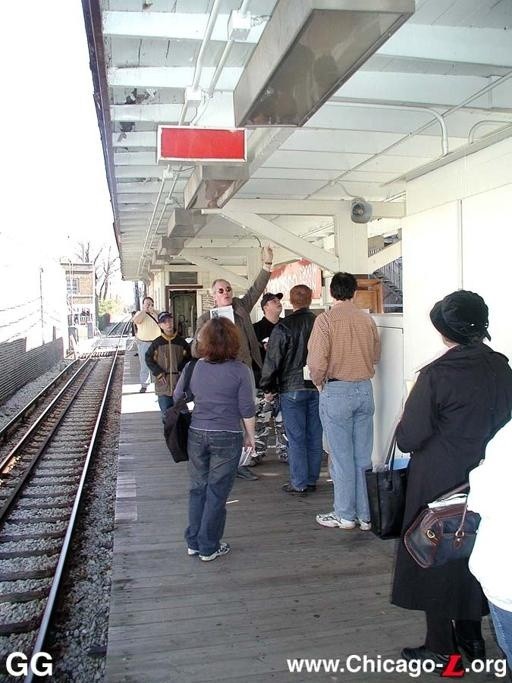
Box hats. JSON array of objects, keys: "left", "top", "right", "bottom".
[
  {"left": 430, "top": 288, "right": 492, "bottom": 344},
  {"left": 155, "top": 311, "right": 174, "bottom": 324},
  {"left": 261, "top": 290, "right": 284, "bottom": 311}
]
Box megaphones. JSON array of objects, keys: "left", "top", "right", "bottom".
[{"left": 351, "top": 198, "right": 372, "bottom": 224}]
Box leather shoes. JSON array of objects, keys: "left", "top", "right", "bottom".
[
  {"left": 455, "top": 634, "right": 488, "bottom": 666},
  {"left": 307, "top": 485, "right": 317, "bottom": 491},
  {"left": 400, "top": 644, "right": 466, "bottom": 679},
  {"left": 236, "top": 465, "right": 260, "bottom": 481},
  {"left": 281, "top": 485, "right": 306, "bottom": 495}
]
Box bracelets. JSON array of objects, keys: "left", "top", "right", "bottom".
[{"left": 264, "top": 261, "right": 272, "bottom": 265}]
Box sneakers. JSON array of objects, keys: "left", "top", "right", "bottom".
[
  {"left": 200, "top": 542, "right": 232, "bottom": 561},
  {"left": 186, "top": 547, "right": 198, "bottom": 555},
  {"left": 139, "top": 387, "right": 147, "bottom": 393},
  {"left": 356, "top": 518, "right": 371, "bottom": 531},
  {"left": 314, "top": 512, "right": 356, "bottom": 530}
]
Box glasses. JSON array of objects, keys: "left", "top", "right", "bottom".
[{"left": 214, "top": 285, "right": 233, "bottom": 294}]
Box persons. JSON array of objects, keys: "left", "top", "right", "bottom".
[
  {"left": 191, "top": 242, "right": 273, "bottom": 481},
  {"left": 248, "top": 292, "right": 288, "bottom": 466},
  {"left": 389, "top": 289, "right": 512, "bottom": 675},
  {"left": 259, "top": 284, "right": 324, "bottom": 493},
  {"left": 466, "top": 417, "right": 511, "bottom": 671},
  {"left": 133, "top": 297, "right": 161, "bottom": 393},
  {"left": 306, "top": 272, "right": 382, "bottom": 531},
  {"left": 178, "top": 314, "right": 187, "bottom": 336},
  {"left": 173, "top": 317, "right": 257, "bottom": 562},
  {"left": 145, "top": 311, "right": 192, "bottom": 423}
]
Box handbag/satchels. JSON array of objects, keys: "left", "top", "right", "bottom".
[
  {"left": 161, "top": 410, "right": 194, "bottom": 465},
  {"left": 363, "top": 457, "right": 411, "bottom": 540},
  {"left": 401, "top": 501, "right": 484, "bottom": 572}
]
